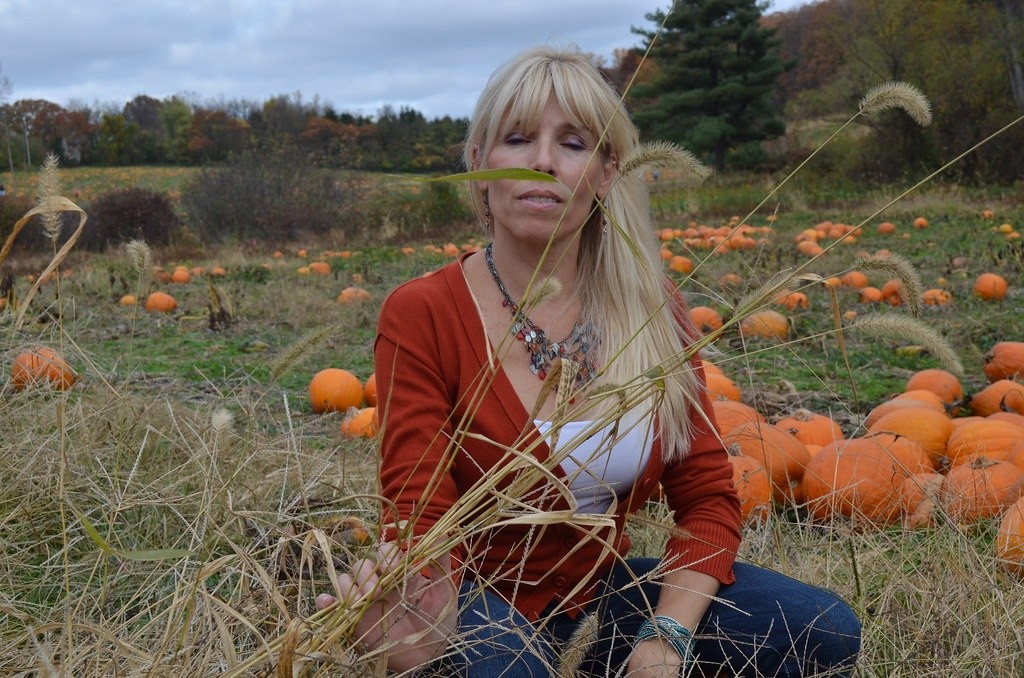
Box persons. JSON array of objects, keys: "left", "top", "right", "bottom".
[{"left": 313, "top": 42, "right": 866, "bottom": 678}]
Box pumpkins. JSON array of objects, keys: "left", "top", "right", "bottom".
[{"left": 0, "top": 210, "right": 1024, "bottom": 577}]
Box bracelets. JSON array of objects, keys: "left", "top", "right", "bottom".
[{"left": 634, "top": 614, "right": 696, "bottom": 669}]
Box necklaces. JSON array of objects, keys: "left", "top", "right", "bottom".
[{"left": 483, "top": 239, "right": 604, "bottom": 407}]
